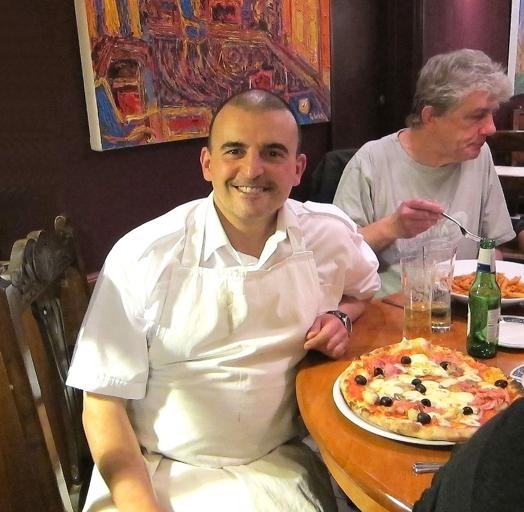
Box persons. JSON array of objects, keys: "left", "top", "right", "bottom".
[
  {"left": 410, "top": 395, "right": 524, "bottom": 511},
  {"left": 328, "top": 45, "right": 516, "bottom": 317},
  {"left": 62, "top": 86, "right": 383, "bottom": 512}
]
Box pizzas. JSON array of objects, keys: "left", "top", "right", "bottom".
[{"left": 339, "top": 337, "right": 523, "bottom": 441}]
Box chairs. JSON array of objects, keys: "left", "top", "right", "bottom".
[
  {"left": 5, "top": 215, "right": 95, "bottom": 511},
  {"left": 310, "top": 148, "right": 361, "bottom": 204}
]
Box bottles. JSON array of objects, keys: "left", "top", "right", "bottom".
[{"left": 466, "top": 238, "right": 501, "bottom": 360}]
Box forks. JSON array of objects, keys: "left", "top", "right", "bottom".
[{"left": 440, "top": 212, "right": 484, "bottom": 242}]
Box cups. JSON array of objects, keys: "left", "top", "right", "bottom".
[
  {"left": 422, "top": 239, "right": 457, "bottom": 331},
  {"left": 399, "top": 255, "right": 435, "bottom": 345}
]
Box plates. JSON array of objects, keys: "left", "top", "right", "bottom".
[
  {"left": 332, "top": 348, "right": 516, "bottom": 446},
  {"left": 497, "top": 314, "right": 524, "bottom": 349},
  {"left": 430, "top": 259, "right": 524, "bottom": 308}
]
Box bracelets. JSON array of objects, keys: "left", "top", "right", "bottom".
[{"left": 325, "top": 307, "right": 353, "bottom": 338}]
